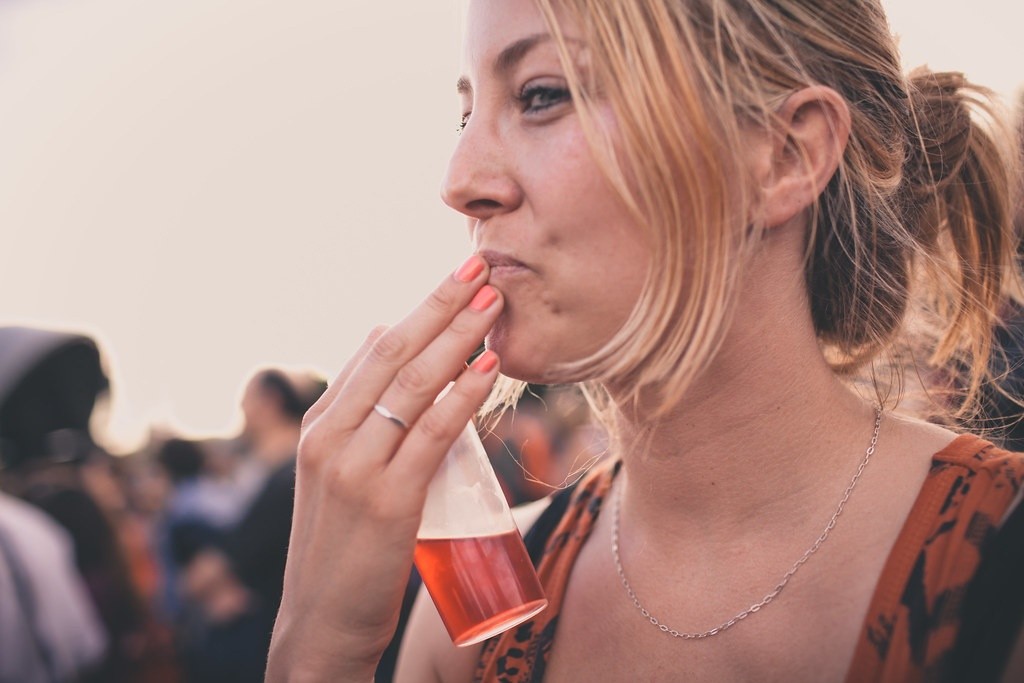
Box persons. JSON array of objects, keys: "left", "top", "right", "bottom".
[
  {"left": 265, "top": 0, "right": 1024, "bottom": 683},
  {"left": 0, "top": 327, "right": 325, "bottom": 683}
]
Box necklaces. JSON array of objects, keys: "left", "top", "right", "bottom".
[{"left": 612, "top": 399, "right": 883, "bottom": 639}]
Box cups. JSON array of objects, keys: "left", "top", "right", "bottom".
[{"left": 411, "top": 380, "right": 548, "bottom": 648}]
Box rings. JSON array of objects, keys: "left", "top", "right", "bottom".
[{"left": 373, "top": 405, "right": 411, "bottom": 430}]
